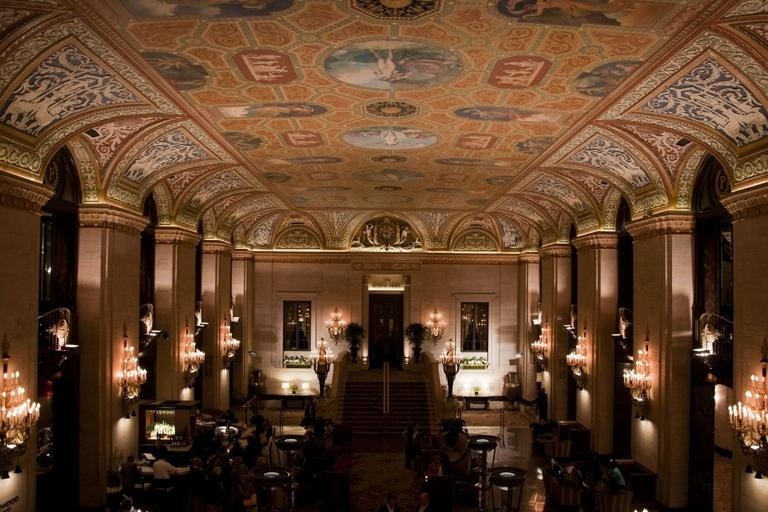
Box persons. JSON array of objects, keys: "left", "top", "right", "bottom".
[
  {"left": 401, "top": 387, "right": 626, "bottom": 511},
  {"left": 125, "top": 408, "right": 335, "bottom": 510},
  {"left": 361, "top": 222, "right": 375, "bottom": 247},
  {"left": 379, "top": 494, "right": 400, "bottom": 511},
  {"left": 398, "top": 226, "right": 411, "bottom": 245}
]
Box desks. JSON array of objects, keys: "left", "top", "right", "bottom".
[
  {"left": 454, "top": 390, "right": 508, "bottom": 410},
  {"left": 247, "top": 464, "right": 293, "bottom": 511},
  {"left": 259, "top": 387, "right": 320, "bottom": 409},
  {"left": 468, "top": 434, "right": 528, "bottom": 512},
  {"left": 275, "top": 434, "right": 305, "bottom": 467}
]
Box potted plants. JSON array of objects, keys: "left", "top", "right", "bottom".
[
  {"left": 344, "top": 321, "right": 365, "bottom": 363},
  {"left": 405, "top": 323, "right": 426, "bottom": 360}
]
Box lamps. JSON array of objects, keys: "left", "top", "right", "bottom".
[
  {"left": 565, "top": 337, "right": 588, "bottom": 389},
  {"left": 324, "top": 306, "right": 345, "bottom": 343},
  {"left": 184, "top": 326, "right": 206, "bottom": 385},
  {"left": 441, "top": 336, "right": 460, "bottom": 398},
  {"left": 122, "top": 331, "right": 147, "bottom": 419},
  {"left": 696, "top": 312, "right": 734, "bottom": 358},
  {"left": 38, "top": 307, "right": 82, "bottom": 355},
  {"left": 0, "top": 332, "right": 43, "bottom": 478},
  {"left": 620, "top": 334, "right": 652, "bottom": 421},
  {"left": 725, "top": 352, "right": 767, "bottom": 481},
  {"left": 425, "top": 308, "right": 447, "bottom": 344},
  {"left": 312, "top": 337, "right": 334, "bottom": 397},
  {"left": 530, "top": 328, "right": 548, "bottom": 373},
  {"left": 222, "top": 314, "right": 242, "bottom": 370}
]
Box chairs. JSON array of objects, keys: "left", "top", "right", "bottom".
[{"left": 531, "top": 420, "right": 658, "bottom": 512}]
